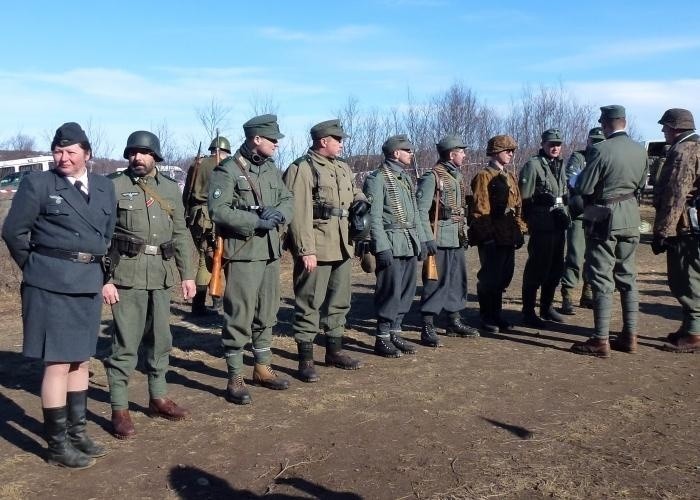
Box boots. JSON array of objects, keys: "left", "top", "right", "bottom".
[
  {"left": 420, "top": 318, "right": 442, "bottom": 349},
  {"left": 251, "top": 361, "right": 292, "bottom": 391},
  {"left": 66, "top": 389, "right": 112, "bottom": 458},
  {"left": 191, "top": 284, "right": 219, "bottom": 316},
  {"left": 225, "top": 374, "right": 253, "bottom": 406},
  {"left": 40, "top": 400, "right": 97, "bottom": 471},
  {"left": 561, "top": 297, "right": 578, "bottom": 316},
  {"left": 446, "top": 317, "right": 481, "bottom": 339}
]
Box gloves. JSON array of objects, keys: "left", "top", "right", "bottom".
[
  {"left": 417, "top": 240, "right": 429, "bottom": 261},
  {"left": 378, "top": 248, "right": 394, "bottom": 267},
  {"left": 351, "top": 200, "right": 370, "bottom": 217},
  {"left": 650, "top": 233, "right": 668, "bottom": 255},
  {"left": 250, "top": 206, "right": 285, "bottom": 230},
  {"left": 426, "top": 239, "right": 439, "bottom": 257}
]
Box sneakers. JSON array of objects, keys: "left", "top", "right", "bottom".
[
  {"left": 540, "top": 304, "right": 569, "bottom": 325},
  {"left": 211, "top": 295, "right": 224, "bottom": 311},
  {"left": 579, "top": 295, "right": 595, "bottom": 309},
  {"left": 519, "top": 309, "right": 547, "bottom": 330}
]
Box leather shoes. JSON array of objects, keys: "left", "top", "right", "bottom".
[
  {"left": 109, "top": 406, "right": 139, "bottom": 442},
  {"left": 662, "top": 326, "right": 700, "bottom": 356},
  {"left": 324, "top": 350, "right": 366, "bottom": 371},
  {"left": 610, "top": 334, "right": 639, "bottom": 355},
  {"left": 389, "top": 332, "right": 418, "bottom": 355},
  {"left": 373, "top": 338, "right": 404, "bottom": 360},
  {"left": 298, "top": 360, "right": 321, "bottom": 384},
  {"left": 480, "top": 314, "right": 515, "bottom": 335},
  {"left": 570, "top": 336, "right": 612, "bottom": 358},
  {"left": 147, "top": 395, "right": 192, "bottom": 423}
]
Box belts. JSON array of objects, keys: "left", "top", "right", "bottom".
[
  {"left": 504, "top": 207, "right": 518, "bottom": 215},
  {"left": 329, "top": 207, "right": 351, "bottom": 217},
  {"left": 451, "top": 207, "right": 464, "bottom": 214},
  {"left": 585, "top": 191, "right": 636, "bottom": 205},
  {"left": 552, "top": 195, "right": 566, "bottom": 205},
  {"left": 192, "top": 200, "right": 209, "bottom": 207},
  {"left": 139, "top": 242, "right": 164, "bottom": 256},
  {"left": 27, "top": 241, "right": 108, "bottom": 266},
  {"left": 382, "top": 221, "right": 416, "bottom": 230}
]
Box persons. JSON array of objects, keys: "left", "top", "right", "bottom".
[
  {"left": 182, "top": 137, "right": 232, "bottom": 316},
  {"left": 520, "top": 105, "right": 649, "bottom": 359},
  {"left": 169, "top": 465, "right": 363, "bottom": 500},
  {"left": 357, "top": 134, "right": 416, "bottom": 358},
  {"left": 413, "top": 135, "right": 479, "bottom": 346},
  {"left": 1, "top": 123, "right": 117, "bottom": 473},
  {"left": 102, "top": 130, "right": 197, "bottom": 440},
  {"left": 465, "top": 134, "right": 528, "bottom": 332},
  {"left": 651, "top": 108, "right": 700, "bottom": 354},
  {"left": 208, "top": 114, "right": 295, "bottom": 406},
  {"left": 282, "top": 119, "right": 371, "bottom": 382}
]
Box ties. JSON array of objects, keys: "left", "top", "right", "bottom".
[{"left": 73, "top": 180, "right": 91, "bottom": 206}]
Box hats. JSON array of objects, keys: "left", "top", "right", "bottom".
[
  {"left": 597, "top": 104, "right": 626, "bottom": 123},
  {"left": 309, "top": 118, "right": 351, "bottom": 141},
  {"left": 588, "top": 126, "right": 607, "bottom": 140},
  {"left": 52, "top": 121, "right": 89, "bottom": 147},
  {"left": 382, "top": 133, "right": 417, "bottom": 157},
  {"left": 541, "top": 127, "right": 564, "bottom": 143},
  {"left": 242, "top": 114, "right": 286, "bottom": 140},
  {"left": 436, "top": 135, "right": 469, "bottom": 153}
]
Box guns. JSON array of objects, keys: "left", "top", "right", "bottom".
[
  {"left": 184, "top": 140, "right": 203, "bottom": 218},
  {"left": 412, "top": 152, "right": 440, "bottom": 281},
  {"left": 209, "top": 128, "right": 223, "bottom": 299}
]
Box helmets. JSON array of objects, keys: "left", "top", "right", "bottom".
[
  {"left": 123, "top": 129, "right": 165, "bottom": 163},
  {"left": 484, "top": 134, "right": 520, "bottom": 155},
  {"left": 656, "top": 108, "right": 697, "bottom": 131},
  {"left": 661, "top": 144, "right": 672, "bottom": 154},
  {"left": 207, "top": 136, "right": 233, "bottom": 154}
]
